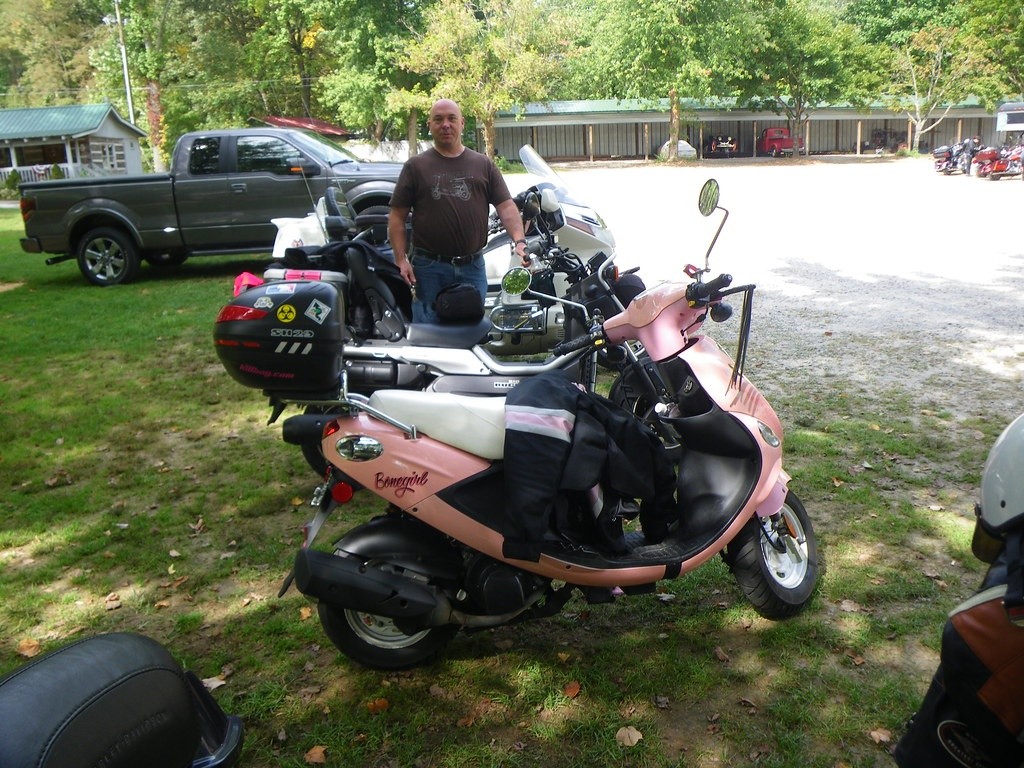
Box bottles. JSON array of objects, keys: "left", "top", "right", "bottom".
[
  {"left": 530, "top": 254, "right": 544, "bottom": 271},
  {"left": 654, "top": 403, "right": 676, "bottom": 417}
]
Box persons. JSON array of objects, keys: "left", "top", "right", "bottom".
[
  {"left": 388, "top": 99, "right": 532, "bottom": 326},
  {"left": 895, "top": 411, "right": 1024, "bottom": 767},
  {"left": 965, "top": 136, "right": 984, "bottom": 176}
]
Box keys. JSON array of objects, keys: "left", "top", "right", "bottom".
[{"left": 412, "top": 289, "right": 416, "bottom": 303}]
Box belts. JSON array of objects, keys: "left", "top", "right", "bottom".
[{"left": 413, "top": 248, "right": 483, "bottom": 267}]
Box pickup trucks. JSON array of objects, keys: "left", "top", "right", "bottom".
[
  {"left": 17, "top": 127, "right": 411, "bottom": 286},
  {"left": 756, "top": 128, "right": 804, "bottom": 157}
]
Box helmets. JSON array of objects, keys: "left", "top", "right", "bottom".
[
  {"left": 970, "top": 412, "right": 1024, "bottom": 539},
  {"left": 434, "top": 282, "right": 485, "bottom": 326}
]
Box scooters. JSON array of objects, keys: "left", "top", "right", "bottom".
[
  {"left": 933, "top": 130, "right": 1024, "bottom": 181},
  {"left": 214, "top": 178, "right": 816, "bottom": 672},
  {"left": 234, "top": 145, "right": 684, "bottom": 462}
]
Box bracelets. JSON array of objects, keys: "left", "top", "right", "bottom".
[{"left": 515, "top": 239, "right": 528, "bottom": 246}]
